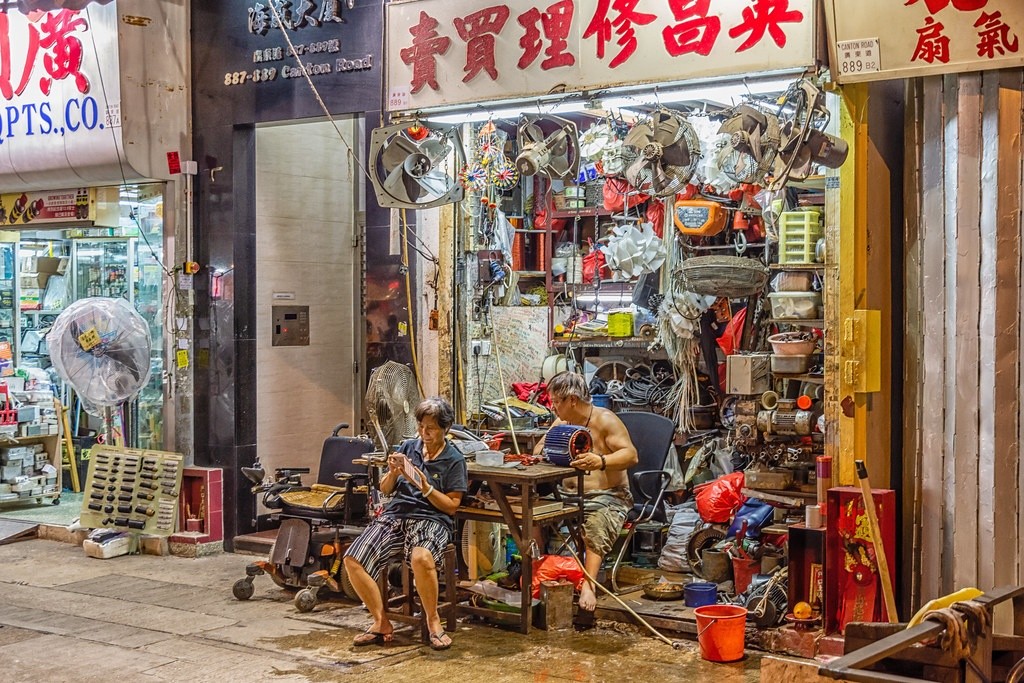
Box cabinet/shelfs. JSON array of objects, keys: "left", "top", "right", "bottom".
[
  {"left": 0, "top": 398, "right": 62, "bottom": 504},
  {"left": 755, "top": 192, "right": 827, "bottom": 497}
]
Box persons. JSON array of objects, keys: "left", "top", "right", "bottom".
[
  {"left": 533, "top": 370, "right": 639, "bottom": 612},
  {"left": 342, "top": 396, "right": 468, "bottom": 651}
]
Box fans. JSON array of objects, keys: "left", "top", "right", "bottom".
[{"left": 60, "top": 297, "right": 152, "bottom": 445}]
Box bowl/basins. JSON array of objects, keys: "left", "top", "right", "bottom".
[
  {"left": 475, "top": 450, "right": 505, "bottom": 467},
  {"left": 642, "top": 583, "right": 683, "bottom": 599},
  {"left": 767, "top": 332, "right": 815, "bottom": 355}
]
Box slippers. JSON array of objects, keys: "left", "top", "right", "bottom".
[
  {"left": 354, "top": 626, "right": 393, "bottom": 646},
  {"left": 429, "top": 631, "right": 453, "bottom": 649}
]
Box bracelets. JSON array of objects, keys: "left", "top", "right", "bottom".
[
  {"left": 596, "top": 453, "right": 606, "bottom": 471},
  {"left": 423, "top": 485, "right": 434, "bottom": 498}
]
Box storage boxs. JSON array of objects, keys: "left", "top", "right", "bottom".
[
  {"left": 20, "top": 256, "right": 61, "bottom": 309},
  {"left": 768, "top": 291, "right": 822, "bottom": 319}
]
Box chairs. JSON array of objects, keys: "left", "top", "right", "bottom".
[{"left": 611, "top": 412, "right": 675, "bottom": 595}]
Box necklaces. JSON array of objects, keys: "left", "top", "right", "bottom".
[{"left": 569, "top": 404, "right": 593, "bottom": 428}]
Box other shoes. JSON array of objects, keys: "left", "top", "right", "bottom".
[{"left": 495, "top": 554, "right": 522, "bottom": 589}]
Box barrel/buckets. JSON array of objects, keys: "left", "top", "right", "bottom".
[
  {"left": 693, "top": 605, "right": 748, "bottom": 662},
  {"left": 733, "top": 555, "right": 761, "bottom": 596}
]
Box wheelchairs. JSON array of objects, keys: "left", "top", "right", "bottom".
[{"left": 232, "top": 423, "right": 388, "bottom": 613}]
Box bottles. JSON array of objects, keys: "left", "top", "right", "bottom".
[{"left": 87, "top": 267, "right": 126, "bottom": 298}]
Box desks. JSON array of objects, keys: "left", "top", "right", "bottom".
[{"left": 438, "top": 463, "right": 585, "bottom": 635}]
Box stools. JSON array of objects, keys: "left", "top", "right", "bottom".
[{"left": 378, "top": 544, "right": 457, "bottom": 643}]
